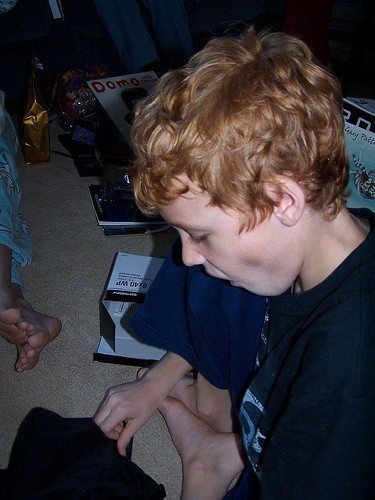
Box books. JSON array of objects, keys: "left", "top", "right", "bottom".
[
  {"left": 87, "top": 168, "right": 170, "bottom": 237},
  {"left": 329, "top": 0, "right": 366, "bottom": 61},
  {"left": 86, "top": 70, "right": 159, "bottom": 148}
]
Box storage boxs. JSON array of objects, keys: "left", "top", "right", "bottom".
[
  {"left": 93, "top": 251, "right": 167, "bottom": 368},
  {"left": 340, "top": 98, "right": 375, "bottom": 212}
]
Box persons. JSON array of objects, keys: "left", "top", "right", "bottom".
[
  {"left": 0, "top": 92, "right": 63, "bottom": 373},
  {"left": 284, "top": 0, "right": 336, "bottom": 69},
  {"left": 93, "top": 0, "right": 194, "bottom": 75},
  {"left": 0, "top": 30, "right": 374, "bottom": 500}
]
